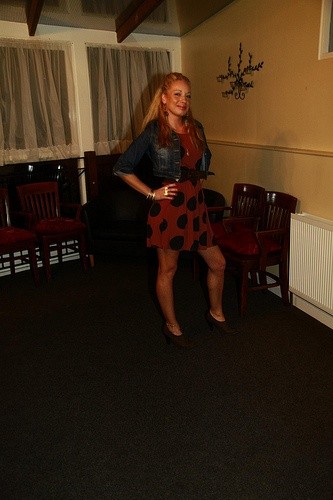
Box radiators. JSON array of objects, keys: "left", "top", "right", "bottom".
[{"left": 289, "top": 213, "right": 333, "bottom": 329}]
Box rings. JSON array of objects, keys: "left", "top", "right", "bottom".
[{"left": 165, "top": 187, "right": 168, "bottom": 197}]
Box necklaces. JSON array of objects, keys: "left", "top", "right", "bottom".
[{"left": 166, "top": 115, "right": 194, "bottom": 158}]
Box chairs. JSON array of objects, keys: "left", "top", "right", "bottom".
[
  {"left": 0, "top": 181, "right": 89, "bottom": 287},
  {"left": 193, "top": 183, "right": 297, "bottom": 315}
]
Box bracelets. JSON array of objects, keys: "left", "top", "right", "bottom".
[{"left": 146, "top": 187, "right": 155, "bottom": 200}]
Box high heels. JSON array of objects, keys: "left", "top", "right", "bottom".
[
  {"left": 161, "top": 322, "right": 189, "bottom": 347},
  {"left": 207, "top": 310, "right": 235, "bottom": 336}
]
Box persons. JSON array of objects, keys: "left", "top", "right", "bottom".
[{"left": 113, "top": 72, "right": 234, "bottom": 346}]
